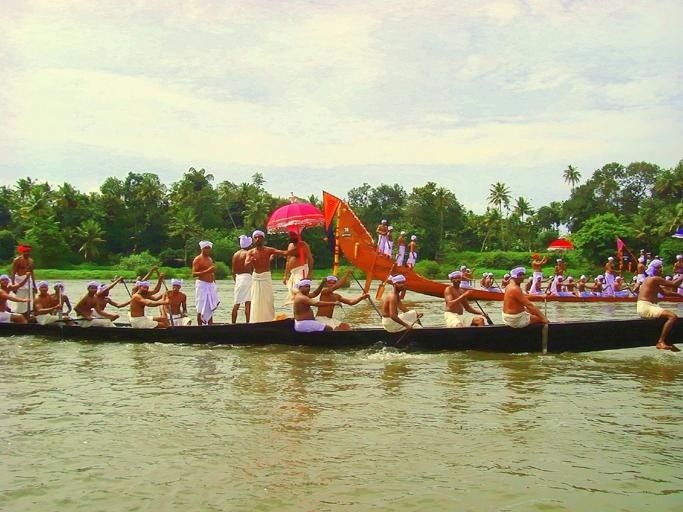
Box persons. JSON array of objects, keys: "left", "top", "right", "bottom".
[
  {"left": 502, "top": 267, "right": 549, "bottom": 328},
  {"left": 396, "top": 231, "right": 406, "bottom": 266},
  {"left": 73, "top": 266, "right": 191, "bottom": 328},
  {"left": 283, "top": 225, "right": 314, "bottom": 306},
  {"left": 381, "top": 272, "right": 423, "bottom": 333},
  {"left": 443, "top": 271, "right": 485, "bottom": 328},
  {"left": 0, "top": 245, "right": 71, "bottom": 324},
  {"left": 384, "top": 225, "right": 393, "bottom": 259},
  {"left": 293, "top": 270, "right": 371, "bottom": 332},
  {"left": 231, "top": 230, "right": 295, "bottom": 323},
  {"left": 375, "top": 219, "right": 388, "bottom": 252},
  {"left": 405, "top": 235, "right": 417, "bottom": 270},
  {"left": 192, "top": 240, "right": 220, "bottom": 326},
  {"left": 459, "top": 249, "right": 682, "bottom": 298},
  {"left": 636, "top": 260, "right": 682, "bottom": 351}
]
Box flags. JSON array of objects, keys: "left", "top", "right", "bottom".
[{"left": 616, "top": 239, "right": 624, "bottom": 269}]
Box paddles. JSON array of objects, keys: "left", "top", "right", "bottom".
[
  {"left": 26, "top": 274, "right": 31, "bottom": 320},
  {"left": 58, "top": 287, "right": 64, "bottom": 339},
  {"left": 540, "top": 297, "right": 549, "bottom": 354},
  {"left": 471, "top": 296, "right": 493, "bottom": 324},
  {"left": 166, "top": 290, "right": 174, "bottom": 327},
  {"left": 56, "top": 317, "right": 105, "bottom": 323}
]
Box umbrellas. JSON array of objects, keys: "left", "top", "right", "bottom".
[
  {"left": 265, "top": 191, "right": 324, "bottom": 266},
  {"left": 544, "top": 237, "right": 575, "bottom": 258}
]
